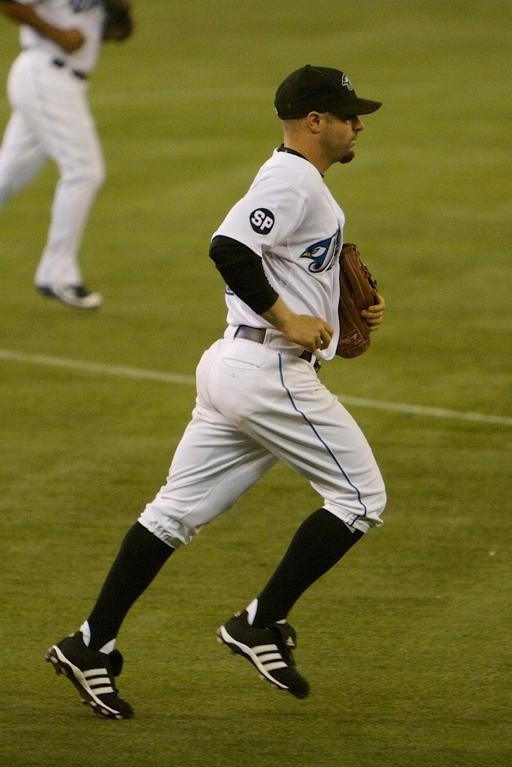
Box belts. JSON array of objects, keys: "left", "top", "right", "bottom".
[
  {"left": 54, "top": 60, "right": 85, "bottom": 79},
  {"left": 234, "top": 325, "right": 321, "bottom": 372}
]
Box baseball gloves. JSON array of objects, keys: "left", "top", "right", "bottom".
[{"left": 336, "top": 243, "right": 377, "bottom": 358}]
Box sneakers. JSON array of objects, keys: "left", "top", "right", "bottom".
[
  {"left": 216, "top": 609, "right": 309, "bottom": 699},
  {"left": 45, "top": 631, "right": 134, "bottom": 720},
  {"left": 40, "top": 285, "right": 102, "bottom": 308}
]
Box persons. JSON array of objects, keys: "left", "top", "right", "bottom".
[
  {"left": 0, "top": 0, "right": 132, "bottom": 308},
  {"left": 42, "top": 64, "right": 387, "bottom": 723}
]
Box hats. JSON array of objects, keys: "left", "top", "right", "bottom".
[{"left": 275, "top": 65, "right": 382, "bottom": 119}]
